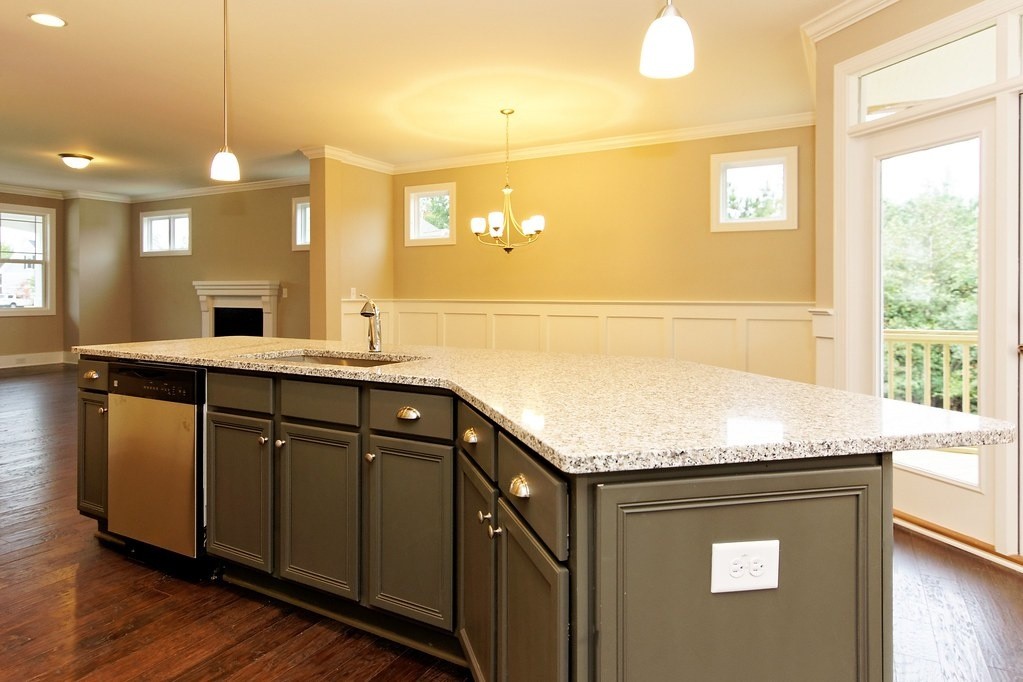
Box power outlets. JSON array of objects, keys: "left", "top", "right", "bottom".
[{"left": 711, "top": 540, "right": 780, "bottom": 595}]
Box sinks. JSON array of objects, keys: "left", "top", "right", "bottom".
[{"left": 259, "top": 354, "right": 413, "bottom": 369}]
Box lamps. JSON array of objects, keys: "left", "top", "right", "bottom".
[
  {"left": 471, "top": 108, "right": 545, "bottom": 255},
  {"left": 640, "top": 0, "right": 695, "bottom": 80},
  {"left": 210, "top": 0, "right": 243, "bottom": 182},
  {"left": 57, "top": 153, "right": 94, "bottom": 170}
]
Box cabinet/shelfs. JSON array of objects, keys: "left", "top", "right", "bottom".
[
  {"left": 455, "top": 400, "right": 894, "bottom": 682},
  {"left": 73, "top": 358, "right": 455, "bottom": 666}
]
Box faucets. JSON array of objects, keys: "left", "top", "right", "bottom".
[{"left": 359, "top": 293, "right": 383, "bottom": 353}]
